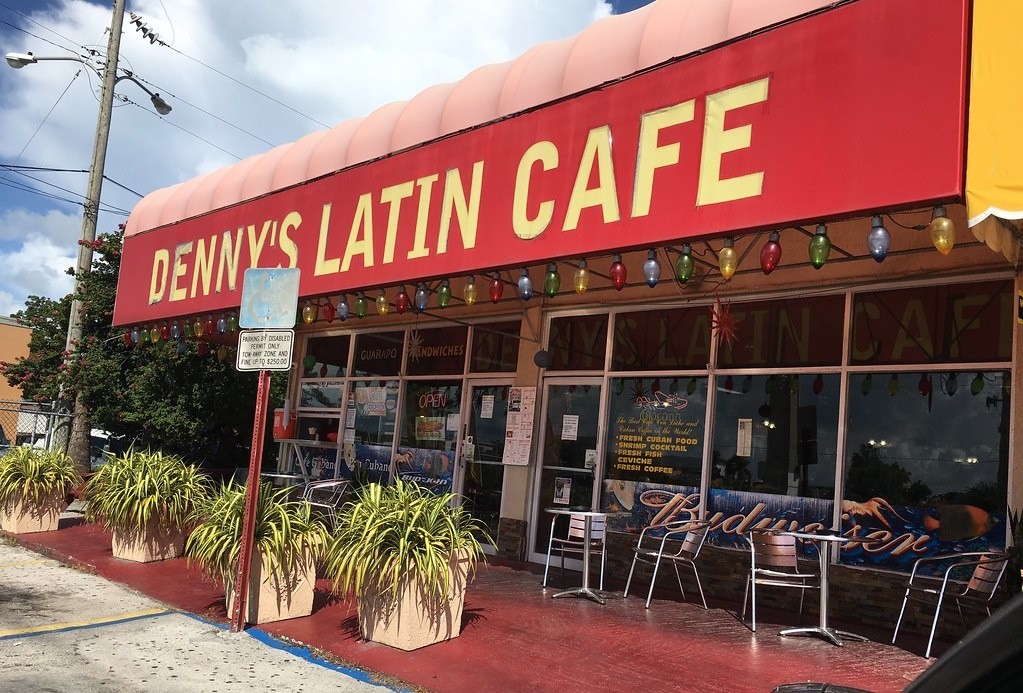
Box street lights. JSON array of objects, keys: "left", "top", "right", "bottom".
[{"left": 5, "top": 47, "right": 173, "bottom": 479}]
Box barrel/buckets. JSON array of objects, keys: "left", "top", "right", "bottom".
[{"left": 273, "top": 408, "right": 298, "bottom": 439}]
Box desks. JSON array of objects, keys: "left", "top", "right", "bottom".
[
  {"left": 778, "top": 533, "right": 883, "bottom": 647},
  {"left": 543, "top": 507, "right": 632, "bottom": 605},
  {"left": 259, "top": 473, "right": 304, "bottom": 504}
]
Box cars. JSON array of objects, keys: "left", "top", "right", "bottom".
[
  {"left": 87, "top": 429, "right": 141, "bottom": 474},
  {"left": 771, "top": 590, "right": 1022, "bottom": 691}
]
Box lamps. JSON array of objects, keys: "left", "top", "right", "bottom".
[{"left": 535, "top": 349, "right": 550, "bottom": 368}]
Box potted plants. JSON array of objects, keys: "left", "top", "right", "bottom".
[
  {"left": 76, "top": 438, "right": 215, "bottom": 563},
  {"left": 324, "top": 473, "right": 499, "bottom": 653},
  {"left": 180, "top": 470, "right": 336, "bottom": 624},
  {"left": 0, "top": 444, "right": 84, "bottom": 534}
]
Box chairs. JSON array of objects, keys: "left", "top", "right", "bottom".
[
  {"left": 543, "top": 513, "right": 609, "bottom": 591},
  {"left": 892, "top": 551, "right": 1010, "bottom": 658},
  {"left": 742, "top": 532, "right": 819, "bottom": 631},
  {"left": 623, "top": 520, "right": 712, "bottom": 610},
  {"left": 297, "top": 478, "right": 350, "bottom": 529}
]
